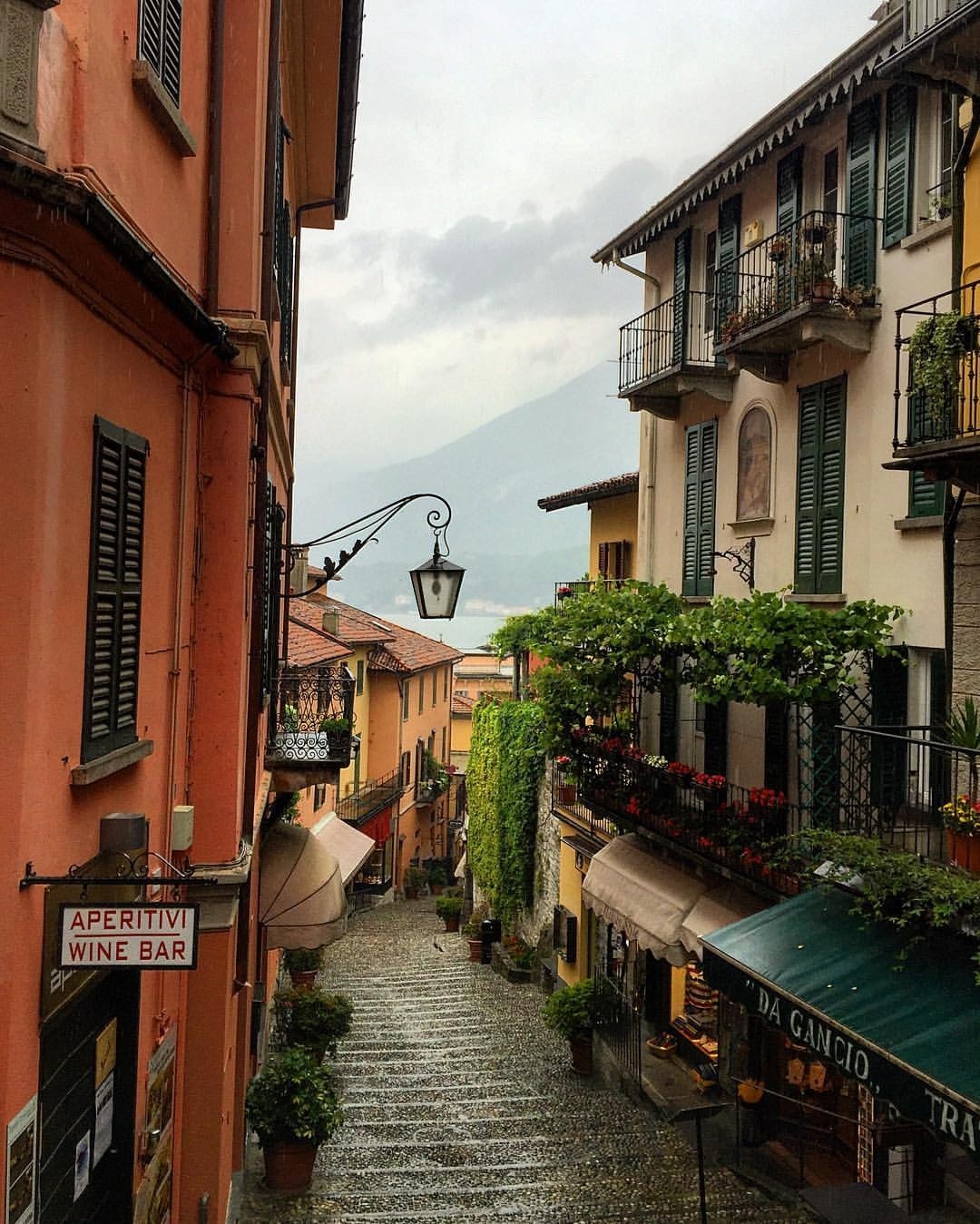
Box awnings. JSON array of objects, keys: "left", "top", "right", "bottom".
[
  {"left": 308, "top": 811, "right": 376, "bottom": 886},
  {"left": 258, "top": 821, "right": 350, "bottom": 952},
  {"left": 580, "top": 833, "right": 776, "bottom": 969},
  {"left": 695, "top": 882, "right": 980, "bottom": 1164}
]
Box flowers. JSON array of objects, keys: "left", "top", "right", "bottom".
[
  {"left": 556, "top": 728, "right": 789, "bottom": 878},
  {"left": 419, "top": 779, "right": 433, "bottom": 792},
  {"left": 933, "top": 693, "right": 980, "bottom": 839}
]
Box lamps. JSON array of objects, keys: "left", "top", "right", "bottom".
[{"left": 272, "top": 494, "right": 466, "bottom": 621}]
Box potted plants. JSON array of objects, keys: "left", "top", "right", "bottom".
[
  {"left": 245, "top": 947, "right": 357, "bottom": 1190},
  {"left": 317, "top": 719, "right": 361, "bottom": 755},
  {"left": 902, "top": 308, "right": 980, "bottom": 438},
  {"left": 763, "top": 827, "right": 980, "bottom": 987},
  {"left": 403, "top": 864, "right": 633, "bottom": 1073},
  {"left": 768, "top": 194, "right": 952, "bottom": 309}
]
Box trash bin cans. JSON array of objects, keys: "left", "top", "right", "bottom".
[{"left": 481, "top": 919, "right": 501, "bottom": 965}]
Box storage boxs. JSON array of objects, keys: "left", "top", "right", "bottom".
[{"left": 646, "top": 1035, "right": 677, "bottom": 1060}]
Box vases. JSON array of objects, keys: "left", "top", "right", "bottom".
[
  {"left": 709, "top": 843, "right": 740, "bottom": 873},
  {"left": 556, "top": 762, "right": 571, "bottom": 773},
  {"left": 605, "top": 753, "right": 625, "bottom": 764},
  {"left": 945, "top": 826, "right": 980, "bottom": 877},
  {"left": 666, "top": 771, "right": 690, "bottom": 790},
  {"left": 421, "top": 789, "right": 432, "bottom": 799},
  {"left": 606, "top": 797, "right": 620, "bottom": 811},
  {"left": 631, "top": 758, "right": 659, "bottom": 778},
  {"left": 631, "top": 807, "right": 653, "bottom": 831},
  {"left": 558, "top": 783, "right": 576, "bottom": 806},
  {"left": 619, "top": 805, "right": 637, "bottom": 821},
  {"left": 691, "top": 780, "right": 725, "bottom": 804},
  {"left": 682, "top": 829, "right": 710, "bottom": 856},
  {"left": 651, "top": 815, "right": 671, "bottom": 838},
  {"left": 773, "top": 872, "right": 810, "bottom": 896},
  {"left": 747, "top": 798, "right": 781, "bottom": 820},
  {"left": 668, "top": 826, "right": 682, "bottom": 844},
  {"left": 744, "top": 860, "right": 775, "bottom": 888}
]
